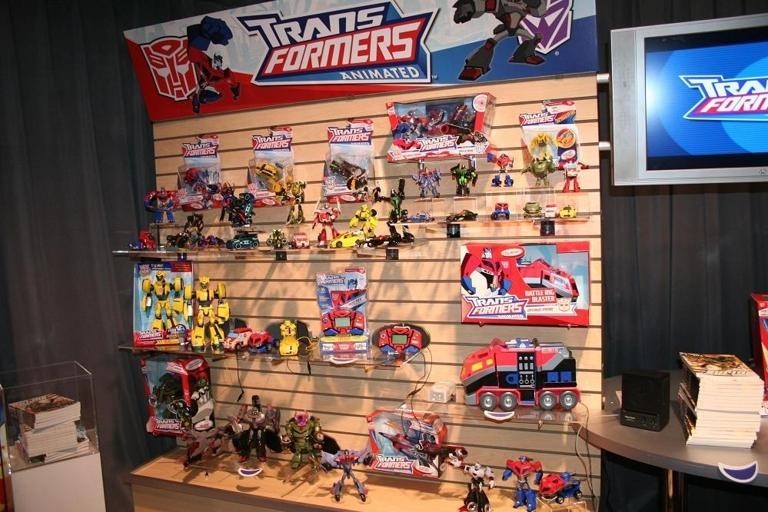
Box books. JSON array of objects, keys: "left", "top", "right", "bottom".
[
  {"left": 675, "top": 350, "right": 768, "bottom": 451},
  {"left": 6, "top": 391, "right": 92, "bottom": 463}
]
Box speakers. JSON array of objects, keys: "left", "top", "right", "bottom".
[{"left": 620, "top": 370, "right": 670, "bottom": 431}]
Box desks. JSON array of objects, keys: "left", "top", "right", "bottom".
[{"left": 571, "top": 369, "right": 767, "bottom": 512}]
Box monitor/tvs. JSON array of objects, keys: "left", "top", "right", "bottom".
[{"left": 610, "top": 13, "right": 768, "bottom": 187}]
